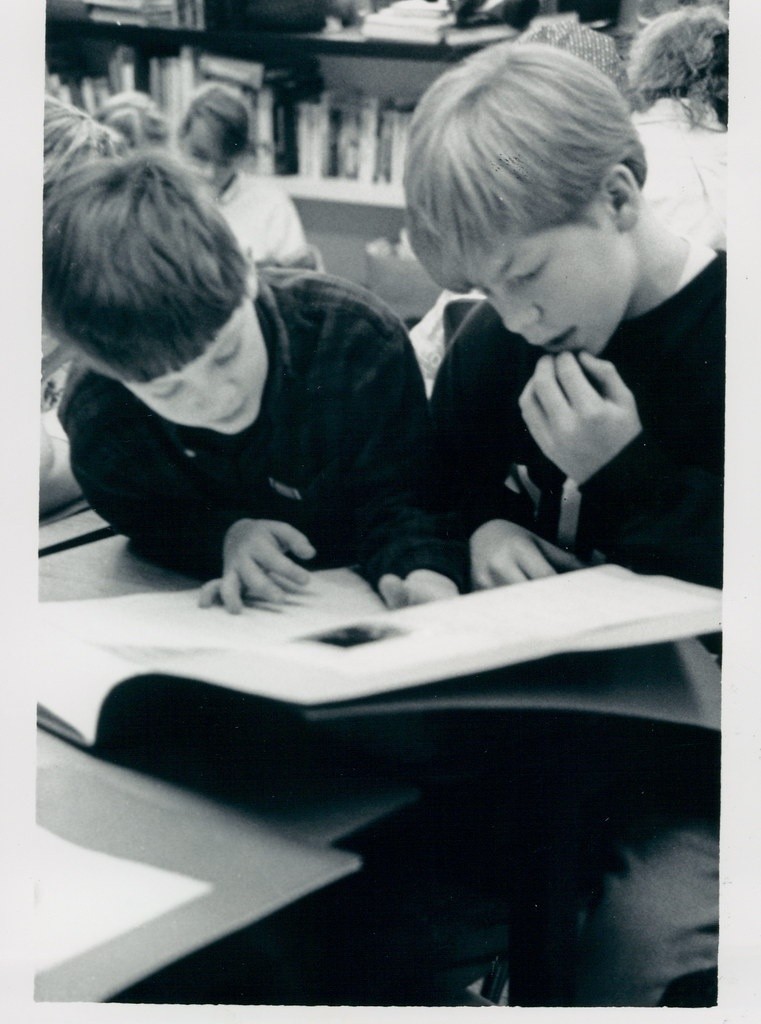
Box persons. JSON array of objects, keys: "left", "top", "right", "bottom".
[
  {"left": 353, "top": 33, "right": 730, "bottom": 1009},
  {"left": 407, "top": 3, "right": 729, "bottom": 401},
  {"left": 41, "top": 85, "right": 319, "bottom": 513},
  {"left": 43, "top": 154, "right": 427, "bottom": 607}
]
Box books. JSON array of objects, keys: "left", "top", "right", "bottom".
[
  {"left": 45, "top": 2, "right": 522, "bottom": 184},
  {"left": 36, "top": 567, "right": 722, "bottom": 776}
]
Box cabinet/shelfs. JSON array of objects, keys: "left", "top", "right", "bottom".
[{"left": 45, "top": 16, "right": 632, "bottom": 210}]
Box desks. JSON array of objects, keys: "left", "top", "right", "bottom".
[{"left": 33, "top": 506, "right": 420, "bottom": 1003}]
